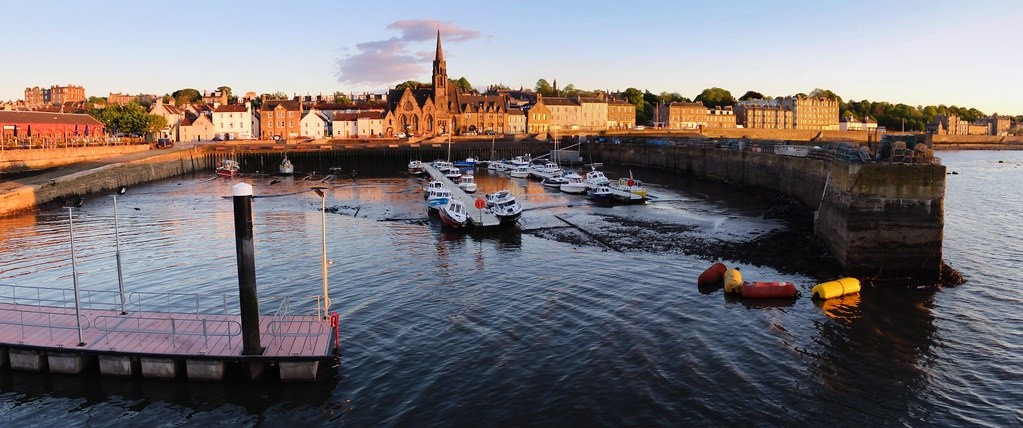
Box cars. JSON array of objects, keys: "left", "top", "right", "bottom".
[
  {"left": 485, "top": 130, "right": 499, "bottom": 136},
  {"left": 635, "top": 125, "right": 644, "bottom": 132}
]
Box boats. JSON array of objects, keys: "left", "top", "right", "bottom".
[
  {"left": 214, "top": 159, "right": 240, "bottom": 179},
  {"left": 407, "top": 123, "right": 648, "bottom": 214},
  {"left": 436, "top": 197, "right": 470, "bottom": 230},
  {"left": 279, "top": 154, "right": 294, "bottom": 176},
  {"left": 484, "top": 188, "right": 523, "bottom": 223}
]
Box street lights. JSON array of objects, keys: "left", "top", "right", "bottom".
[
  {"left": 108, "top": 185, "right": 128, "bottom": 317},
  {"left": 312, "top": 185, "right": 333, "bottom": 317},
  {"left": 62, "top": 197, "right": 86, "bottom": 347}
]
